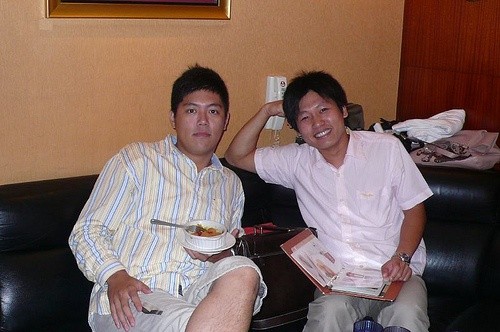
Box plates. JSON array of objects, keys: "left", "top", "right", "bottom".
[{"left": 176, "top": 229, "right": 236, "bottom": 255}]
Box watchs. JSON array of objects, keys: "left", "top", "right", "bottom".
[{"left": 391, "top": 251, "right": 411, "bottom": 265}]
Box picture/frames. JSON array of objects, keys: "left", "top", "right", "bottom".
[{"left": 44, "top": 0, "right": 232, "bottom": 21}]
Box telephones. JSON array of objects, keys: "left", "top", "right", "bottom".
[{"left": 262, "top": 74, "right": 288, "bottom": 150}]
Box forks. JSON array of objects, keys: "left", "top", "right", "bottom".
[{"left": 151, "top": 219, "right": 206, "bottom": 232}]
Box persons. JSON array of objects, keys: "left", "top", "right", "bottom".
[
  {"left": 68, "top": 63, "right": 267, "bottom": 332},
  {"left": 224, "top": 71, "right": 433, "bottom": 332}
]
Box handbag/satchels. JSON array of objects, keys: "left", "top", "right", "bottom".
[
  {"left": 234, "top": 223, "right": 319, "bottom": 321},
  {"left": 367, "top": 117, "right": 425, "bottom": 152},
  {"left": 411, "top": 112, "right": 500, "bottom": 170}
]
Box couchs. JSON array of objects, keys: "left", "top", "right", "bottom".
[{"left": 0, "top": 158, "right": 500, "bottom": 332}]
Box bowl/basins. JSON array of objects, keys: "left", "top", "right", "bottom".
[{"left": 184, "top": 220, "right": 228, "bottom": 249}]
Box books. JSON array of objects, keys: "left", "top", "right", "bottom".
[{"left": 280, "top": 228, "right": 404, "bottom": 301}]
